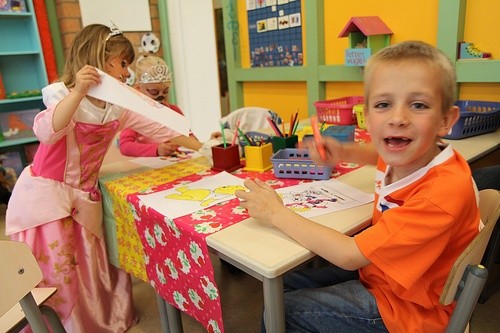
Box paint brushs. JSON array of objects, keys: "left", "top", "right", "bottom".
[
  {"left": 268, "top": 109, "right": 300, "bottom": 138},
  {"left": 220, "top": 120, "right": 270, "bottom": 147},
  {"left": 311, "top": 115, "right": 329, "bottom": 164}
]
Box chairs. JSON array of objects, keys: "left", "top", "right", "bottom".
[
  {"left": 439, "top": 188, "right": 500, "bottom": 333},
  {"left": 0, "top": 240, "right": 68, "bottom": 333}
]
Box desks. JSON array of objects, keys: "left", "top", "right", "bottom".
[{"left": 97, "top": 117, "right": 500, "bottom": 333}]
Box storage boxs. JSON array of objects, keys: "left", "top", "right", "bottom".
[
  {"left": 441, "top": 100, "right": 500, "bottom": 139},
  {"left": 313, "top": 96, "right": 364, "bottom": 125},
  {"left": 271, "top": 147, "right": 333, "bottom": 180}
]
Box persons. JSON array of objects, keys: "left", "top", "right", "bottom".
[
  {"left": 234, "top": 39, "right": 485, "bottom": 333},
  {"left": 6, "top": 22, "right": 221, "bottom": 333},
  {"left": 119, "top": 54, "right": 201, "bottom": 158}
]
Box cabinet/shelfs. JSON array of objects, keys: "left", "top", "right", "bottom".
[{"left": 0, "top": 0, "right": 51, "bottom": 147}]
